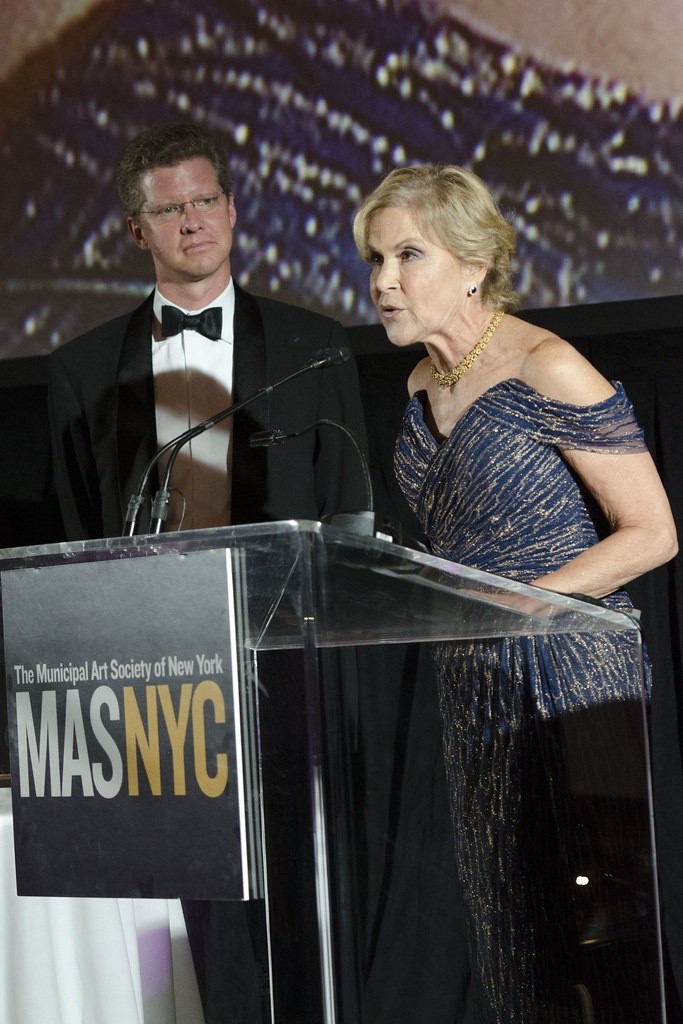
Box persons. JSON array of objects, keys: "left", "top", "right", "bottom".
[
  {"left": 354, "top": 169, "right": 680, "bottom": 1023},
  {"left": 30, "top": 123, "right": 370, "bottom": 541}
]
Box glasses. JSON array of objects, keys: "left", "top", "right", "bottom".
[{"left": 136, "top": 191, "right": 227, "bottom": 222}]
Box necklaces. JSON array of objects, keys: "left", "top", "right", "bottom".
[{"left": 430, "top": 308, "right": 506, "bottom": 388}]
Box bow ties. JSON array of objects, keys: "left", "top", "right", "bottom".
[{"left": 161, "top": 304, "right": 223, "bottom": 341}]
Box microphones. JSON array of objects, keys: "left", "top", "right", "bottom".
[{"left": 122, "top": 345, "right": 375, "bottom": 538}]
